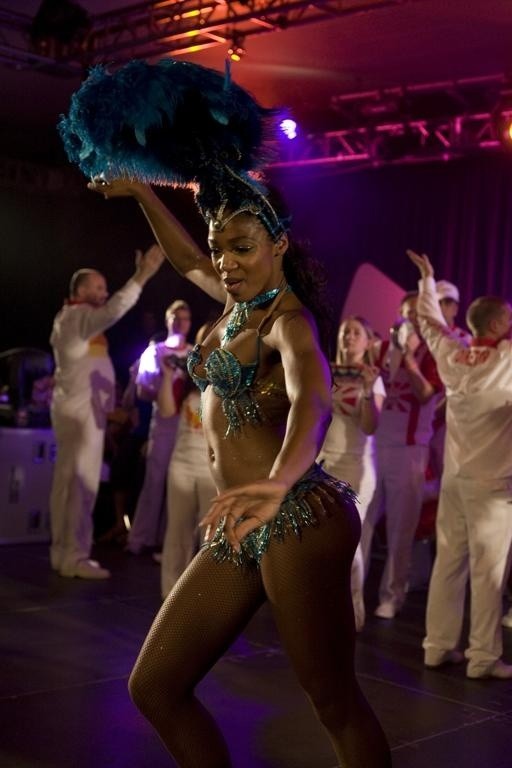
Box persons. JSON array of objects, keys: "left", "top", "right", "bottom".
[
  {"left": 120, "top": 298, "right": 195, "bottom": 553},
  {"left": 47, "top": 241, "right": 167, "bottom": 580},
  {"left": 88, "top": 160, "right": 393, "bottom": 766},
  {"left": 314, "top": 313, "right": 388, "bottom": 633},
  {"left": 435, "top": 278, "right": 472, "bottom": 344},
  {"left": 157, "top": 321, "right": 220, "bottom": 599},
  {"left": 360, "top": 288, "right": 447, "bottom": 618},
  {"left": 405, "top": 247, "right": 512, "bottom": 680}
]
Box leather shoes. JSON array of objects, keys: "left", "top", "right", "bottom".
[
  {"left": 52, "top": 558, "right": 110, "bottom": 579},
  {"left": 468, "top": 659, "right": 512, "bottom": 679},
  {"left": 425, "top": 652, "right": 463, "bottom": 666},
  {"left": 125, "top": 544, "right": 162, "bottom": 567},
  {"left": 374, "top": 604, "right": 399, "bottom": 619}
]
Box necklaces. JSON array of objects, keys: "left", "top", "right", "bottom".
[{"left": 223, "top": 284, "right": 293, "bottom": 340}]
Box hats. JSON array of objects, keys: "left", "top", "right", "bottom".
[{"left": 435, "top": 280, "right": 459, "bottom": 303}]
